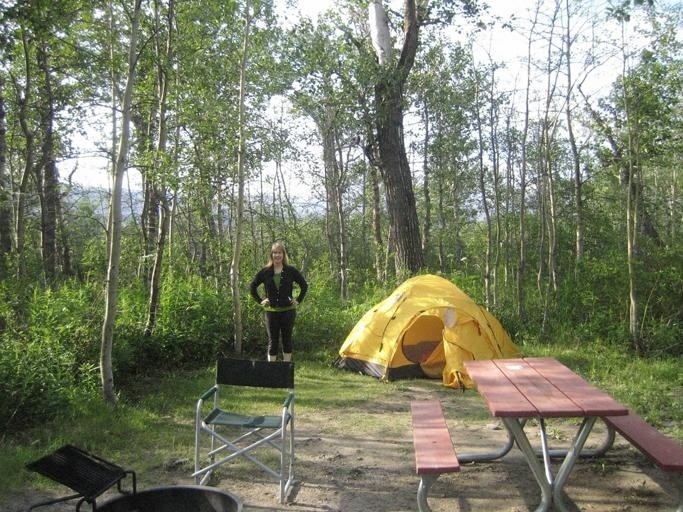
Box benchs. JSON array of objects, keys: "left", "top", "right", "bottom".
[
  {"left": 583, "top": 389, "right": 683, "bottom": 512},
  {"left": 408, "top": 394, "right": 546, "bottom": 512}
]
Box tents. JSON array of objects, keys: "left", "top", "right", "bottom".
[{"left": 332, "top": 272, "right": 520, "bottom": 392}]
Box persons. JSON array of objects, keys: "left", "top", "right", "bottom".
[{"left": 250, "top": 240, "right": 308, "bottom": 362}]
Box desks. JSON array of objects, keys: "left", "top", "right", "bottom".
[{"left": 462, "top": 356, "right": 630, "bottom": 512}]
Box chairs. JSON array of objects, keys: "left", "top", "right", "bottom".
[{"left": 191, "top": 356, "right": 298, "bottom": 505}]
